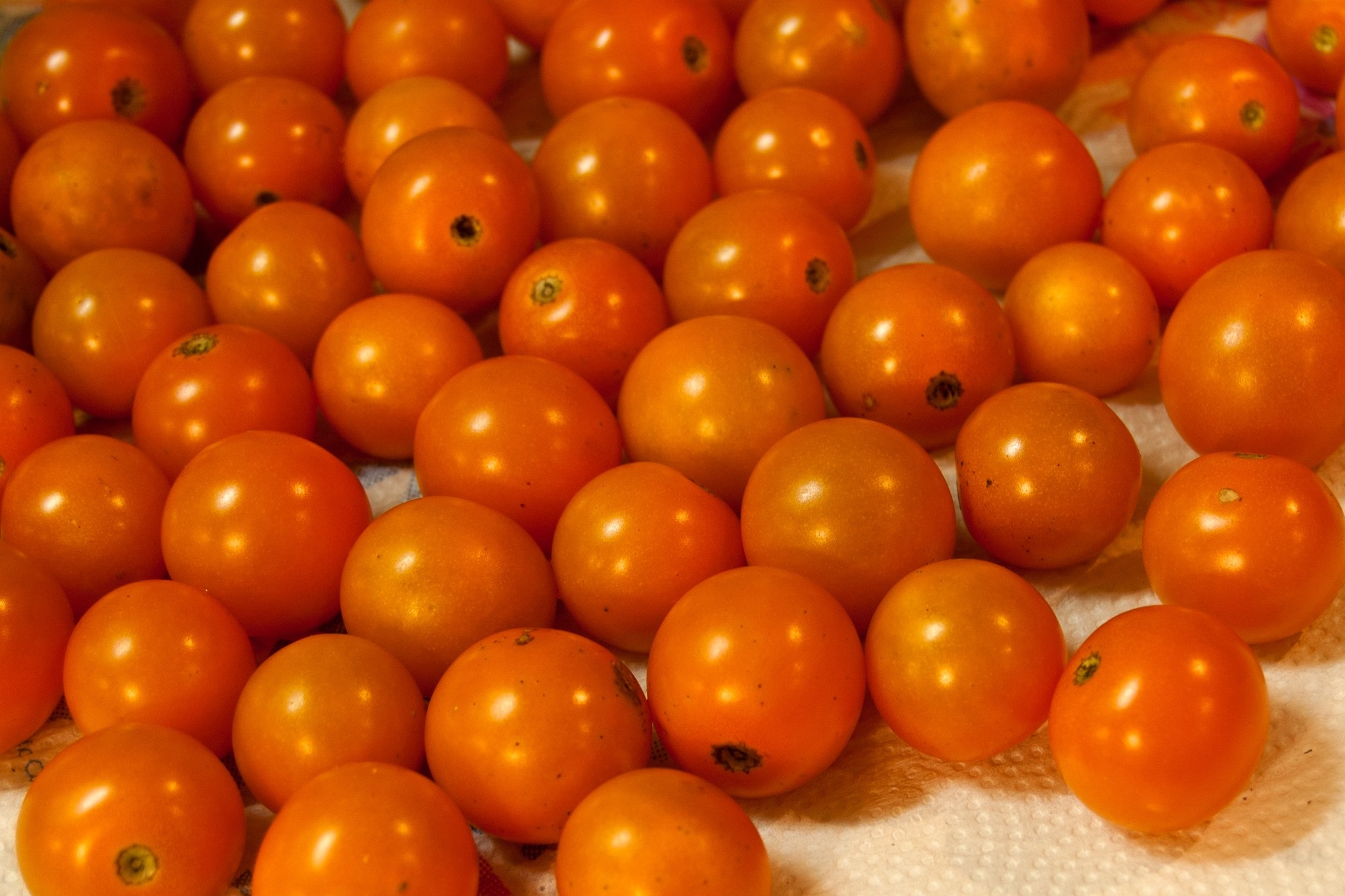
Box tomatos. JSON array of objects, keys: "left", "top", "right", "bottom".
[{"left": 0, "top": 0, "right": 1345, "bottom": 896}]
